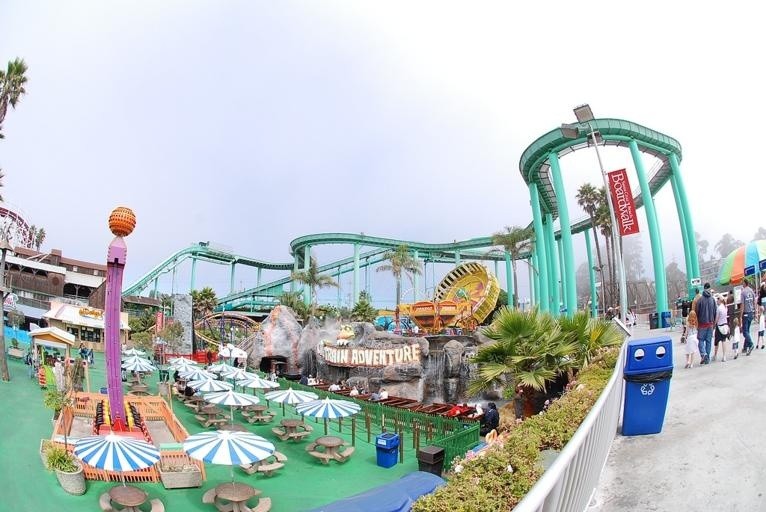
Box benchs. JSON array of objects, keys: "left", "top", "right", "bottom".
[
  {"left": 148, "top": 498, "right": 165, "bottom": 512},
  {"left": 125, "top": 378, "right": 355, "bottom": 477},
  {"left": 99, "top": 492, "right": 113, "bottom": 512},
  {"left": 203, "top": 486, "right": 271, "bottom": 512}
]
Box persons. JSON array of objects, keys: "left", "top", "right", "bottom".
[
  {"left": 692, "top": 288, "right": 703, "bottom": 309},
  {"left": 444, "top": 402, "right": 499, "bottom": 435},
  {"left": 513, "top": 385, "right": 525, "bottom": 425},
  {"left": 328, "top": 382, "right": 341, "bottom": 391},
  {"left": 695, "top": 283, "right": 717, "bottom": 364},
  {"left": 759, "top": 281, "right": 766, "bottom": 297},
  {"left": 378, "top": 387, "right": 389, "bottom": 400},
  {"left": 367, "top": 390, "right": 379, "bottom": 400},
  {"left": 79, "top": 343, "right": 96, "bottom": 368},
  {"left": 713, "top": 294, "right": 731, "bottom": 362},
  {"left": 341, "top": 380, "right": 347, "bottom": 390},
  {"left": 357, "top": 385, "right": 366, "bottom": 395},
  {"left": 685, "top": 310, "right": 699, "bottom": 369},
  {"left": 727, "top": 289, "right": 735, "bottom": 304},
  {"left": 298, "top": 373, "right": 308, "bottom": 385},
  {"left": 605, "top": 300, "right": 638, "bottom": 326},
  {"left": 728, "top": 317, "right": 743, "bottom": 358},
  {"left": 754, "top": 306, "right": 766, "bottom": 349},
  {"left": 675, "top": 298, "right": 693, "bottom": 317},
  {"left": 307, "top": 374, "right": 316, "bottom": 385},
  {"left": 349, "top": 385, "right": 359, "bottom": 396},
  {"left": 26, "top": 345, "right": 64, "bottom": 371},
  {"left": 739, "top": 278, "right": 758, "bottom": 355}
]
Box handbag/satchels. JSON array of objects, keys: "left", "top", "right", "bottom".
[{"left": 718, "top": 325, "right": 730, "bottom": 335}]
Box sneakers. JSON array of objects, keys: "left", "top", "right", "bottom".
[
  {"left": 742, "top": 343, "right": 754, "bottom": 356},
  {"left": 700, "top": 353, "right": 708, "bottom": 364}
]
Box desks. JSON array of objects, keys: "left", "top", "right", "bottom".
[
  {"left": 110, "top": 485, "right": 147, "bottom": 512},
  {"left": 215, "top": 482, "right": 255, "bottom": 512}
]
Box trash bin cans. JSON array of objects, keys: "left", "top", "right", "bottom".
[
  {"left": 649, "top": 312, "right": 671, "bottom": 329},
  {"left": 159, "top": 370, "right": 169, "bottom": 382},
  {"left": 622, "top": 336, "right": 673, "bottom": 436},
  {"left": 375, "top": 432, "right": 400, "bottom": 468},
  {"left": 419, "top": 446, "right": 444, "bottom": 477}
]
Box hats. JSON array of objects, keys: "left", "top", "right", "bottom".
[{"left": 704, "top": 283, "right": 710, "bottom": 289}]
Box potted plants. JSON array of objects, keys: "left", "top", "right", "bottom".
[
  {"left": 38, "top": 388, "right": 86, "bottom": 495},
  {"left": 7, "top": 309, "right": 25, "bottom": 358}
]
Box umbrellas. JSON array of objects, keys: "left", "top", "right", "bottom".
[
  {"left": 182, "top": 430, "right": 275, "bottom": 486},
  {"left": 72, "top": 433, "right": 162, "bottom": 488},
  {"left": 294, "top": 396, "right": 361, "bottom": 435},
  {"left": 715, "top": 238, "right": 766, "bottom": 296},
  {"left": 263, "top": 386, "right": 320, "bottom": 419},
  {"left": 120, "top": 346, "right": 280, "bottom": 429}
]
